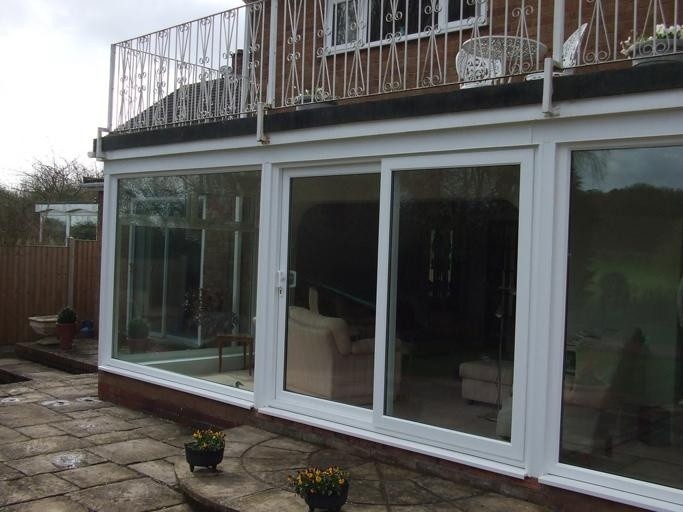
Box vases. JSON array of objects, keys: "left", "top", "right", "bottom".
[
  {"left": 304, "top": 480, "right": 349, "bottom": 511},
  {"left": 186, "top": 441, "right": 224, "bottom": 472},
  {"left": 626, "top": 39, "right": 683, "bottom": 67},
  {"left": 28, "top": 314, "right": 59, "bottom": 346}
]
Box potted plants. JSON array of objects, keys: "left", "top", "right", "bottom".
[
  {"left": 181, "top": 285, "right": 240, "bottom": 349},
  {"left": 125, "top": 318, "right": 150, "bottom": 352},
  {"left": 55, "top": 305, "right": 79, "bottom": 349}
]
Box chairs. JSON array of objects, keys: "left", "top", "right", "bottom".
[
  {"left": 458, "top": 316, "right": 643, "bottom": 434},
  {"left": 456, "top": 47, "right": 504, "bottom": 89},
  {"left": 526, "top": 22, "right": 590, "bottom": 79},
  {"left": 286, "top": 305, "right": 403, "bottom": 406}
]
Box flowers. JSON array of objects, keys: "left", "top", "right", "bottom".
[
  {"left": 617, "top": 22, "right": 683, "bottom": 56},
  {"left": 288, "top": 463, "right": 351, "bottom": 500},
  {"left": 193, "top": 428, "right": 226, "bottom": 452}
]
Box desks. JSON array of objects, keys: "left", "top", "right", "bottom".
[
  {"left": 217, "top": 333, "right": 252, "bottom": 373},
  {"left": 463, "top": 34, "right": 548, "bottom": 84}
]
